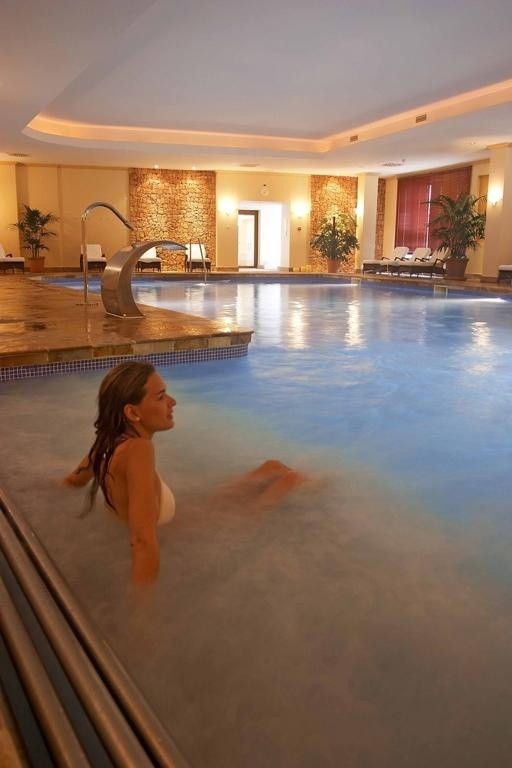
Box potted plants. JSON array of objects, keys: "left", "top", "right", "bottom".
[
  {"left": 309, "top": 213, "right": 360, "bottom": 274},
  {"left": 8, "top": 204, "right": 62, "bottom": 274},
  {"left": 419, "top": 190, "right": 488, "bottom": 281}
]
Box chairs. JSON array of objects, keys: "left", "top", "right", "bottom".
[
  {"left": 80, "top": 244, "right": 107, "bottom": 273},
  {"left": 184, "top": 243, "right": 212, "bottom": 273},
  {"left": 135, "top": 244, "right": 162, "bottom": 272},
  {"left": 362, "top": 246, "right": 450, "bottom": 279},
  {"left": 0, "top": 242, "right": 25, "bottom": 275},
  {"left": 496, "top": 264, "right": 512, "bottom": 284}
]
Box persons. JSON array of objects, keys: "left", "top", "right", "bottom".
[{"left": 64, "top": 360, "right": 314, "bottom": 601}]
